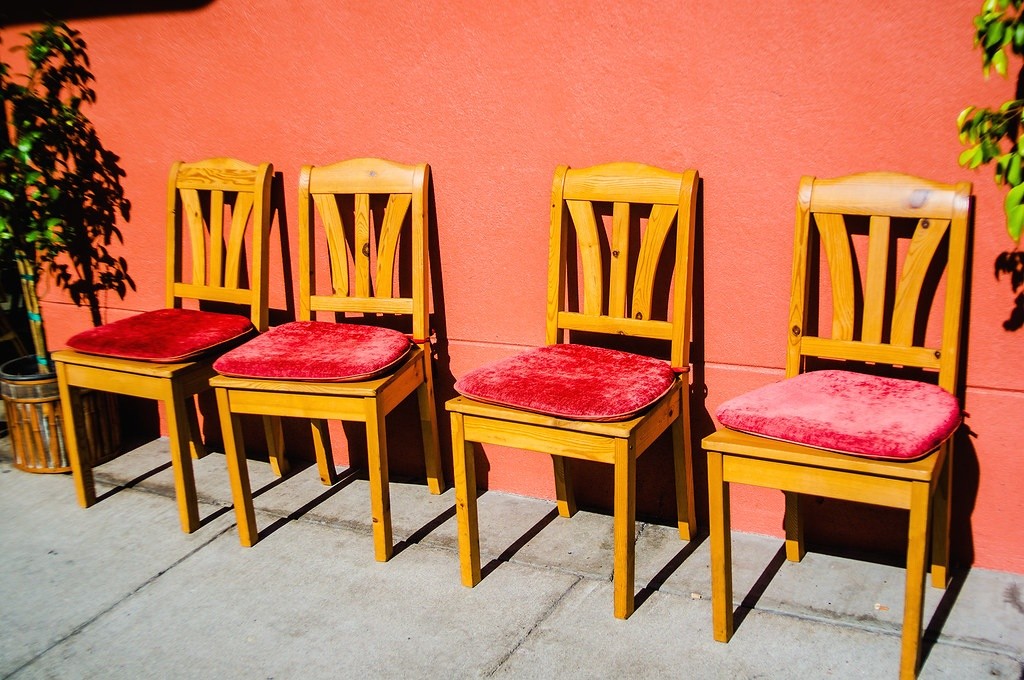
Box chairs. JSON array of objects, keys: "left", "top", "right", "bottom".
[
  {"left": 51, "top": 161, "right": 289, "bottom": 533},
  {"left": 443, "top": 157, "right": 699, "bottom": 620},
  {"left": 208, "top": 156, "right": 444, "bottom": 562},
  {"left": 702, "top": 170, "right": 973, "bottom": 680}
]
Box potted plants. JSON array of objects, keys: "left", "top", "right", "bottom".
[{"left": 0, "top": 20, "right": 121, "bottom": 476}]
